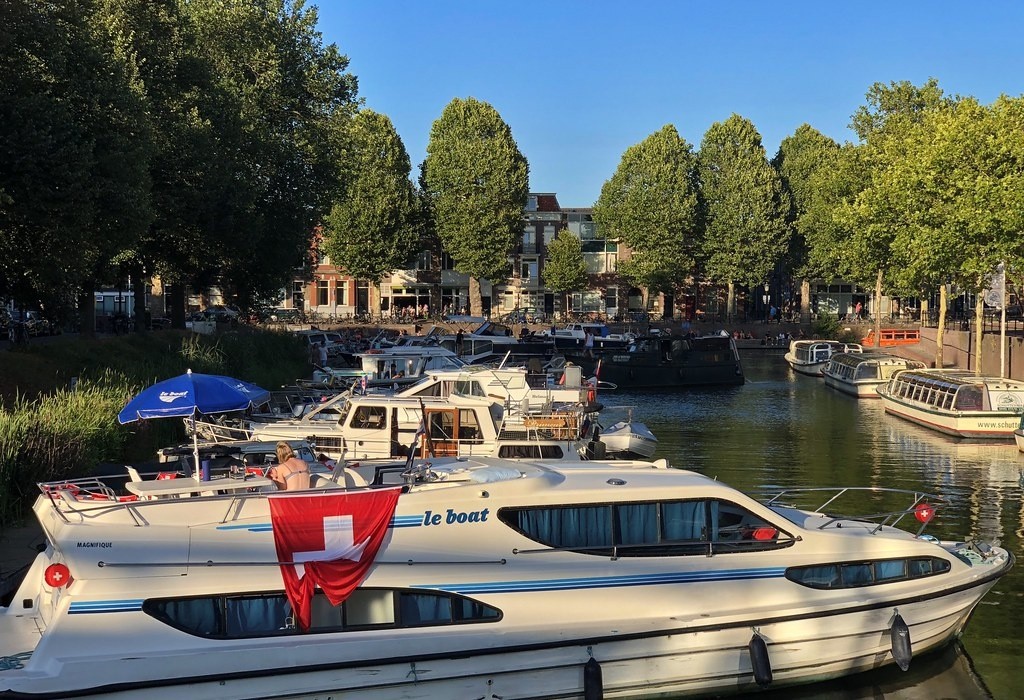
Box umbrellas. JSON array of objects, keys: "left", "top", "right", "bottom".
[{"left": 118, "top": 369, "right": 271, "bottom": 483}]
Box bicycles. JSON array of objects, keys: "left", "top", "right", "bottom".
[
  {"left": 4, "top": 321, "right": 31, "bottom": 355},
  {"left": 250, "top": 310, "right": 405, "bottom": 324}
]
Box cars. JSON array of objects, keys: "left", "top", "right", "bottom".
[
  {"left": 500, "top": 307, "right": 546, "bottom": 324},
  {"left": 202, "top": 305, "right": 240, "bottom": 318}
]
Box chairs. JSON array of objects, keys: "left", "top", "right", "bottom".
[
  {"left": 371, "top": 442, "right": 419, "bottom": 485},
  {"left": 40, "top": 462, "right": 275, "bottom": 503}
]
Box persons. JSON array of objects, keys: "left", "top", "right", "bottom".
[
  {"left": 559, "top": 362, "right": 574, "bottom": 385},
  {"left": 583, "top": 328, "right": 594, "bottom": 358},
  {"left": 310, "top": 340, "right": 328, "bottom": 370},
  {"left": 512, "top": 307, "right": 530, "bottom": 325},
  {"left": 687, "top": 329, "right": 698, "bottom": 338},
  {"left": 733, "top": 329, "right": 807, "bottom": 345},
  {"left": 383, "top": 363, "right": 405, "bottom": 379},
  {"left": 769, "top": 305, "right": 782, "bottom": 326},
  {"left": 391, "top": 304, "right": 466, "bottom": 320},
  {"left": 845, "top": 302, "right": 863, "bottom": 324},
  {"left": 456, "top": 329, "right": 464, "bottom": 358},
  {"left": 270, "top": 441, "right": 310, "bottom": 490}
]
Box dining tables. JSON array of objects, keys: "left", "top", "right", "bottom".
[{"left": 127, "top": 474, "right": 274, "bottom": 497}]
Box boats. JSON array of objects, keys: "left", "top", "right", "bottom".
[
  {"left": 0, "top": 467, "right": 1021, "bottom": 699},
  {"left": 874, "top": 368, "right": 1024, "bottom": 438},
  {"left": 784, "top": 339, "right": 864, "bottom": 377},
  {"left": 860, "top": 328, "right": 921, "bottom": 347},
  {"left": 820, "top": 352, "right": 928, "bottom": 399},
  {"left": 35, "top": 317, "right": 747, "bottom": 543}
]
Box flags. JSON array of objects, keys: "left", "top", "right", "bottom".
[
  {"left": 267, "top": 486, "right": 403, "bottom": 632},
  {"left": 360, "top": 374, "right": 368, "bottom": 395}
]
